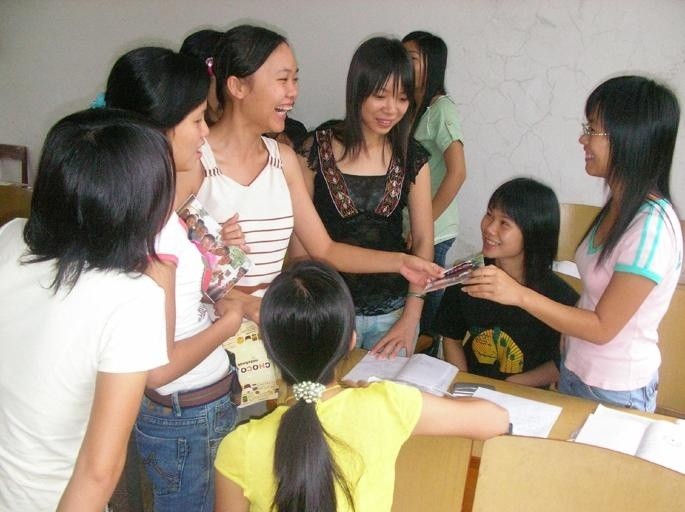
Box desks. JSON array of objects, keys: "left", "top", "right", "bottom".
[{"left": 347, "top": 343, "right": 685, "bottom": 473}]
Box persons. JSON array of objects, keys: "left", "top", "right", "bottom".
[
  {"left": 0, "top": 106, "right": 176, "bottom": 512},
  {"left": 461, "top": 76, "right": 682, "bottom": 414},
  {"left": 432, "top": 177, "right": 580, "bottom": 389},
  {"left": 98, "top": 25, "right": 467, "bottom": 512}
]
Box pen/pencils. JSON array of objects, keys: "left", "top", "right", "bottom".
[{"left": 426, "top": 262, "right": 474, "bottom": 284}]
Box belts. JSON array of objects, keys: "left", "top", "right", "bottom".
[{"left": 146, "top": 366, "right": 242, "bottom": 408}]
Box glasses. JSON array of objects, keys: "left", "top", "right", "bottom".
[{"left": 580, "top": 123, "right": 607, "bottom": 136}]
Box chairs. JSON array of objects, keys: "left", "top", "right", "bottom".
[
  {"left": 556, "top": 201, "right": 605, "bottom": 263},
  {"left": 391, "top": 438, "right": 470, "bottom": 512},
  {"left": 473, "top": 435, "right": 685, "bottom": 512}
]
